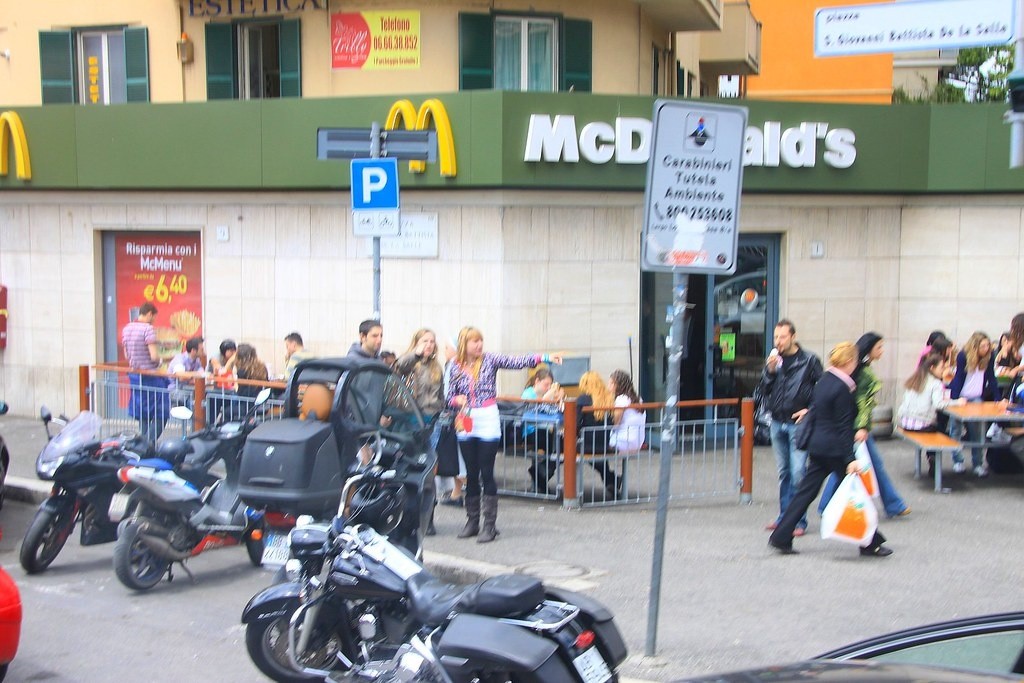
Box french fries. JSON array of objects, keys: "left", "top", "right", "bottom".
[{"left": 170, "top": 309, "right": 202, "bottom": 335}]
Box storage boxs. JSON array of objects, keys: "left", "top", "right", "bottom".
[
  {"left": 237, "top": 417, "right": 339, "bottom": 494},
  {"left": 437, "top": 587, "right": 628, "bottom": 683}
]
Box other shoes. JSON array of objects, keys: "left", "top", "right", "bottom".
[
  {"left": 766, "top": 521, "right": 777, "bottom": 530},
  {"left": 606, "top": 475, "right": 621, "bottom": 500},
  {"left": 439, "top": 491, "right": 463, "bottom": 506},
  {"left": 792, "top": 527, "right": 804, "bottom": 536},
  {"left": 928, "top": 455, "right": 936, "bottom": 479},
  {"left": 768, "top": 539, "right": 798, "bottom": 554},
  {"left": 954, "top": 462, "right": 966, "bottom": 473},
  {"left": 860, "top": 545, "right": 893, "bottom": 556},
  {"left": 973, "top": 465, "right": 988, "bottom": 477},
  {"left": 888, "top": 506, "right": 912, "bottom": 517},
  {"left": 426, "top": 521, "right": 436, "bottom": 536}
]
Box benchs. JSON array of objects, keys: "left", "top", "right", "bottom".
[
  {"left": 508, "top": 442, "right": 654, "bottom": 500},
  {"left": 893, "top": 427, "right": 964, "bottom": 494}
]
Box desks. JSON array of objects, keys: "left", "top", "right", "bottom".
[
  {"left": 183, "top": 383, "right": 233, "bottom": 396},
  {"left": 942, "top": 400, "right": 1024, "bottom": 448}
]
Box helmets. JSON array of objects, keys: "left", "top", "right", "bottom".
[
  {"left": 350, "top": 482, "right": 407, "bottom": 535},
  {"left": 156, "top": 439, "right": 187, "bottom": 466}
]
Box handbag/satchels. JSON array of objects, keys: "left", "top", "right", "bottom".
[
  {"left": 435, "top": 396, "right": 460, "bottom": 476},
  {"left": 795, "top": 405, "right": 816, "bottom": 450},
  {"left": 846, "top": 439, "right": 880, "bottom": 500},
  {"left": 986, "top": 422, "right": 1013, "bottom": 445},
  {"left": 820, "top": 470, "right": 878, "bottom": 548}
]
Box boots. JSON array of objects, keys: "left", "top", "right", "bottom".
[
  {"left": 477, "top": 493, "right": 499, "bottom": 543},
  {"left": 457, "top": 495, "right": 480, "bottom": 538}
]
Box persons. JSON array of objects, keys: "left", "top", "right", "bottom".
[
  {"left": 577, "top": 371, "right": 621, "bottom": 497},
  {"left": 759, "top": 320, "right": 824, "bottom": 537},
  {"left": 608, "top": 369, "right": 645, "bottom": 451},
  {"left": 224, "top": 344, "right": 271, "bottom": 422},
  {"left": 347, "top": 320, "right": 387, "bottom": 515},
  {"left": 817, "top": 333, "right": 911, "bottom": 521},
  {"left": 122, "top": 302, "right": 170, "bottom": 451},
  {"left": 435, "top": 326, "right": 566, "bottom": 543},
  {"left": 283, "top": 332, "right": 316, "bottom": 381},
  {"left": 767, "top": 342, "right": 894, "bottom": 558},
  {"left": 385, "top": 329, "right": 444, "bottom": 536},
  {"left": 381, "top": 351, "right": 396, "bottom": 366},
  {"left": 167, "top": 337, "right": 204, "bottom": 403},
  {"left": 521, "top": 368, "right": 563, "bottom": 495},
  {"left": 897, "top": 331, "right": 967, "bottom": 477},
  {"left": 951, "top": 332, "right": 996, "bottom": 477},
  {"left": 207, "top": 341, "right": 237, "bottom": 374},
  {"left": 994, "top": 313, "right": 1024, "bottom": 401}
]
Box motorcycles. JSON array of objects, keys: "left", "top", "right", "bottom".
[
  {"left": 18, "top": 356, "right": 442, "bottom": 593},
  {"left": 239, "top": 404, "right": 629, "bottom": 683}
]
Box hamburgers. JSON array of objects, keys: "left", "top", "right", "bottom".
[{"left": 155, "top": 328, "right": 182, "bottom": 359}]
